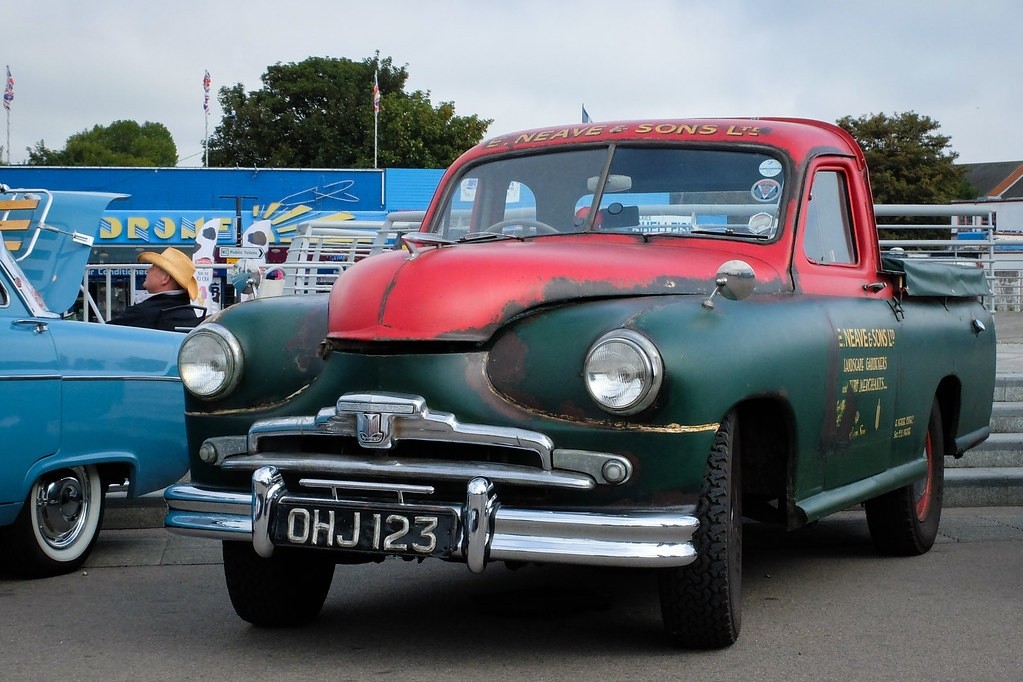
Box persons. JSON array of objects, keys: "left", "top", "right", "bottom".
[{"left": 105, "top": 247, "right": 201, "bottom": 333}]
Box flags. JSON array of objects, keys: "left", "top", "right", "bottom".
[
  {"left": 203, "top": 72, "right": 211, "bottom": 116},
  {"left": 373, "top": 77, "right": 380, "bottom": 113},
  {"left": 3, "top": 69, "right": 15, "bottom": 111},
  {"left": 582, "top": 108, "right": 593, "bottom": 123}
]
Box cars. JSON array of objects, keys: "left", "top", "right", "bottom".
[{"left": 0, "top": 183, "right": 202, "bottom": 579}]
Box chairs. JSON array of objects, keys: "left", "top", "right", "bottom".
[
  {"left": 602, "top": 204, "right": 642, "bottom": 232},
  {"left": 155, "top": 305, "right": 207, "bottom": 332}
]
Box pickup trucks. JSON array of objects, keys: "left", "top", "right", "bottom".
[{"left": 162, "top": 117, "right": 996, "bottom": 652}]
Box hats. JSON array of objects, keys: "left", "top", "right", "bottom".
[{"left": 136, "top": 247, "right": 199, "bottom": 302}]
